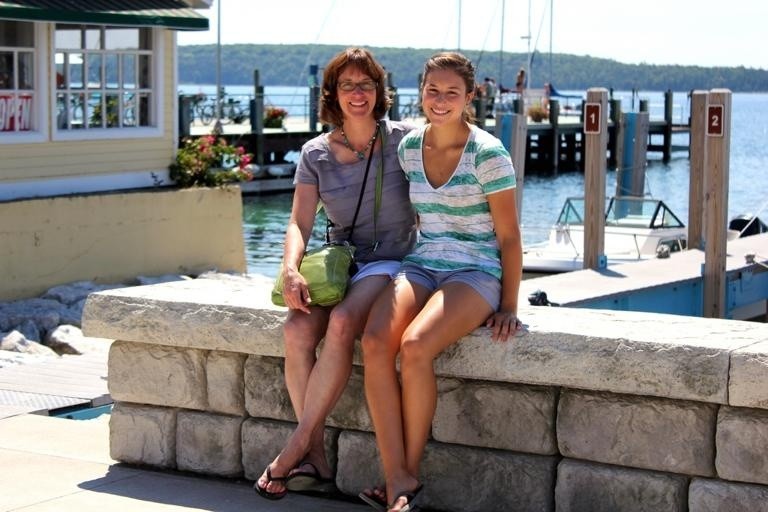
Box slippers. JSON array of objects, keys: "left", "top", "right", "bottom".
[
  {"left": 358, "top": 483, "right": 425, "bottom": 511},
  {"left": 253, "top": 462, "right": 335, "bottom": 500}
]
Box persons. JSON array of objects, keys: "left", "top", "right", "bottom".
[
  {"left": 516, "top": 69, "right": 525, "bottom": 99},
  {"left": 479, "top": 77, "right": 497, "bottom": 116},
  {"left": 359, "top": 50, "right": 522, "bottom": 512},
  {"left": 543, "top": 83, "right": 551, "bottom": 109},
  {"left": 251, "top": 47, "right": 418, "bottom": 499}
]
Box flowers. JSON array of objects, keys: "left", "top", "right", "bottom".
[
  {"left": 167, "top": 137, "right": 254, "bottom": 190},
  {"left": 528, "top": 98, "right": 549, "bottom": 123},
  {"left": 57, "top": 72, "right": 131, "bottom": 128},
  {"left": 263, "top": 107, "right": 289, "bottom": 128}
]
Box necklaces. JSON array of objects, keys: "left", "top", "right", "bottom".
[{"left": 339, "top": 119, "right": 380, "bottom": 161}]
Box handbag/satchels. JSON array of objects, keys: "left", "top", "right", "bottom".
[{"left": 272, "top": 241, "right": 358, "bottom": 308}]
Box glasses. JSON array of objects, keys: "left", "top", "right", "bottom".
[{"left": 337, "top": 80, "right": 377, "bottom": 91}]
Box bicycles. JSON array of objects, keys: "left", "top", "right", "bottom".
[
  {"left": 57, "top": 92, "right": 136, "bottom": 127},
  {"left": 190, "top": 96, "right": 252, "bottom": 125}
]
